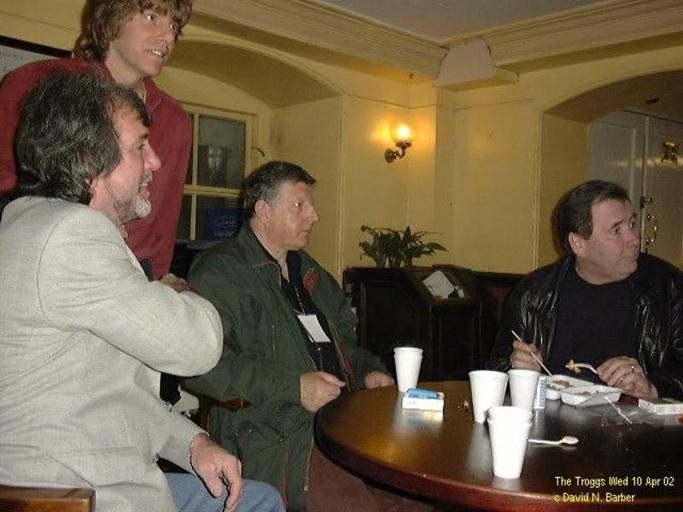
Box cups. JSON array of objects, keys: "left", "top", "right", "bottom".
[
  {"left": 393, "top": 346, "right": 423, "bottom": 393},
  {"left": 468, "top": 368, "right": 541, "bottom": 479},
  {"left": 534, "top": 377, "right": 547, "bottom": 409}
]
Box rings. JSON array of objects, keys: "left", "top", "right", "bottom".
[{"left": 629, "top": 363, "right": 636, "bottom": 373}]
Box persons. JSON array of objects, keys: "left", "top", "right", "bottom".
[
  {"left": 489, "top": 178, "right": 683, "bottom": 400},
  {"left": 0, "top": 0, "right": 193, "bottom": 280},
  {"left": 0, "top": 64, "right": 288, "bottom": 512},
  {"left": 181, "top": 159, "right": 436, "bottom": 511}
]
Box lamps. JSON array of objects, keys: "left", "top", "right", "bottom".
[{"left": 385, "top": 122, "right": 413, "bottom": 163}]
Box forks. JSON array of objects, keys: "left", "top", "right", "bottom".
[{"left": 566, "top": 362, "right": 599, "bottom": 377}]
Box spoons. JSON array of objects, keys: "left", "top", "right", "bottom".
[{"left": 529, "top": 436, "right": 581, "bottom": 448}]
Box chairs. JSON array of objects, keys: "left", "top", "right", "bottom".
[
  {"left": 0, "top": 484, "right": 96, "bottom": 512},
  {"left": 180, "top": 384, "right": 253, "bottom": 437}
]
